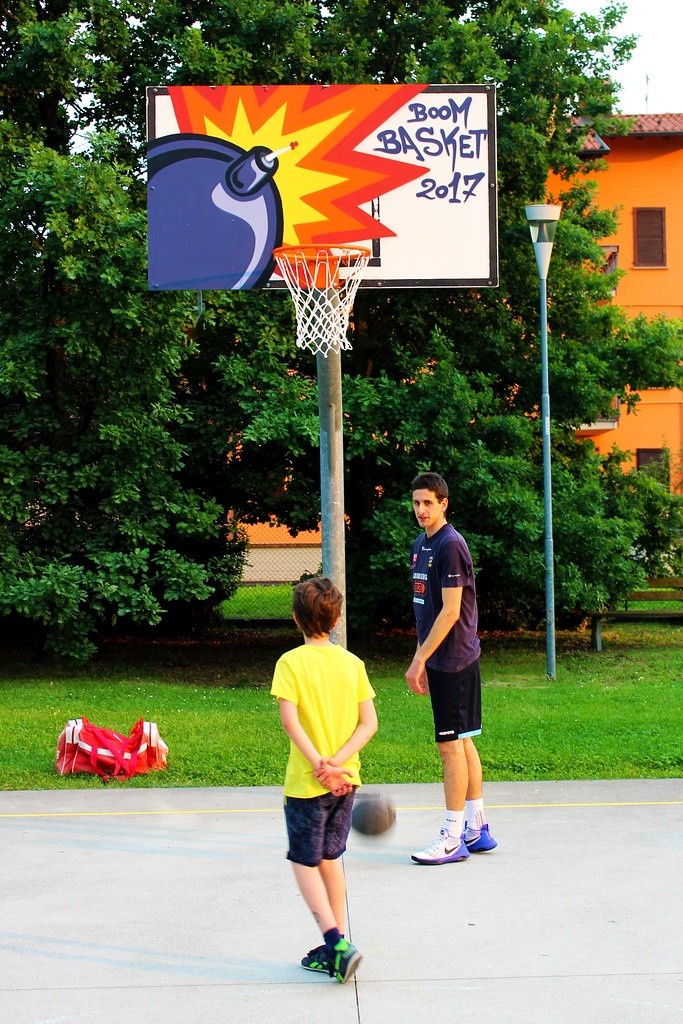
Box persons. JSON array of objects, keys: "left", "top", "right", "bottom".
[
  {"left": 404, "top": 473, "right": 499, "bottom": 865},
  {"left": 270, "top": 576, "right": 377, "bottom": 982}
]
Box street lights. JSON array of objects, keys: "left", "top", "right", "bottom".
[{"left": 524, "top": 202, "right": 566, "bottom": 678}]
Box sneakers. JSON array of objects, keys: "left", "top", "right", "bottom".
[
  {"left": 464, "top": 820, "right": 497, "bottom": 853},
  {"left": 324, "top": 936, "right": 361, "bottom": 984},
  {"left": 411, "top": 828, "right": 471, "bottom": 865},
  {"left": 301, "top": 944, "right": 337, "bottom": 977}
]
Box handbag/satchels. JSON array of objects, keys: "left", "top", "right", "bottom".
[{"left": 55, "top": 716, "right": 170, "bottom": 783}]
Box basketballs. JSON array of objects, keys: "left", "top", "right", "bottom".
[{"left": 352, "top": 799, "right": 397, "bottom": 836}]
